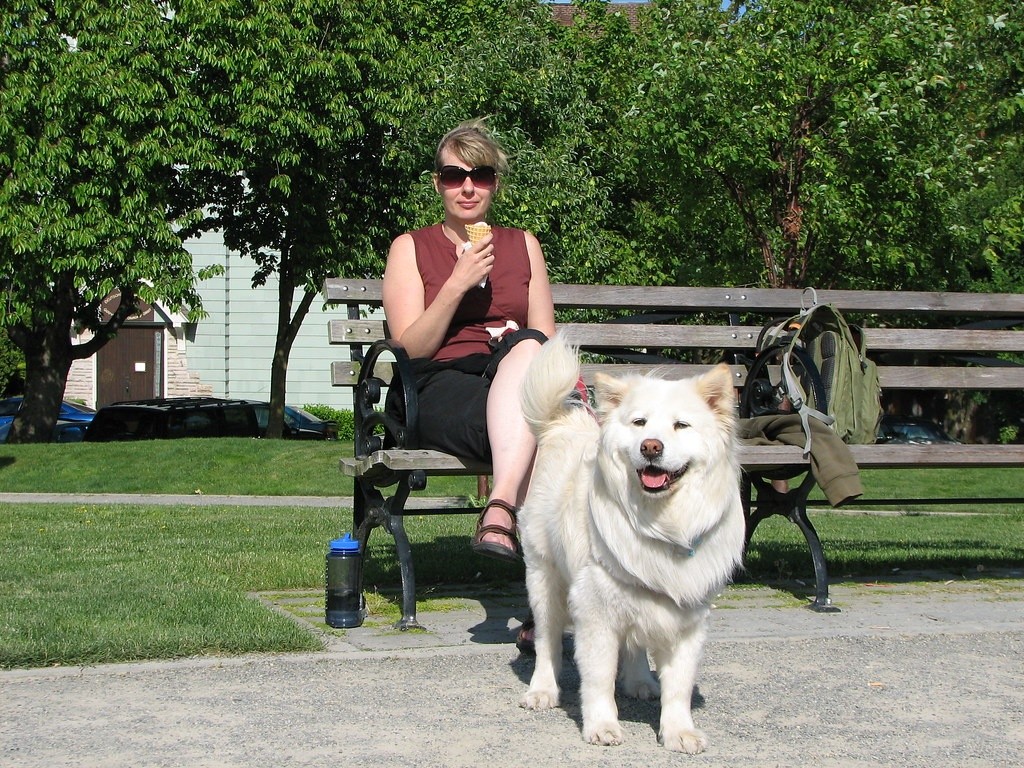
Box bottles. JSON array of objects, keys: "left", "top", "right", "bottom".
[{"left": 325, "top": 532, "right": 363, "bottom": 628}]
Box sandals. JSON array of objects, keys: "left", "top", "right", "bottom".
[
  {"left": 516, "top": 619, "right": 537, "bottom": 649},
  {"left": 469, "top": 499, "right": 523, "bottom": 566}
]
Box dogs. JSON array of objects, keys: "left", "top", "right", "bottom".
[{"left": 512, "top": 329, "right": 747, "bottom": 755}]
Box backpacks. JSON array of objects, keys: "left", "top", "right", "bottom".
[{"left": 774, "top": 304, "right": 883, "bottom": 446}]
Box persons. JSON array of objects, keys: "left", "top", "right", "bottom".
[{"left": 382, "top": 118, "right": 581, "bottom": 650}]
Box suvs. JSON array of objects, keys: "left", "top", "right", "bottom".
[{"left": 83, "top": 397, "right": 325, "bottom": 440}]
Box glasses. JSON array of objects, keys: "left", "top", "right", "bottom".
[{"left": 436, "top": 165, "right": 499, "bottom": 190}]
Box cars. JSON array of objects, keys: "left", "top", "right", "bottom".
[
  {"left": 875, "top": 415, "right": 967, "bottom": 445},
  {"left": 285, "top": 406, "right": 339, "bottom": 440},
  {"left": 0, "top": 397, "right": 98, "bottom": 443}
]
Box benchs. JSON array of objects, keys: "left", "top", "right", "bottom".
[{"left": 322, "top": 277, "right": 1024, "bottom": 630}]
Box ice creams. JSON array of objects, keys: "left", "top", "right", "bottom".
[{"left": 465, "top": 221, "right": 491, "bottom": 244}]
